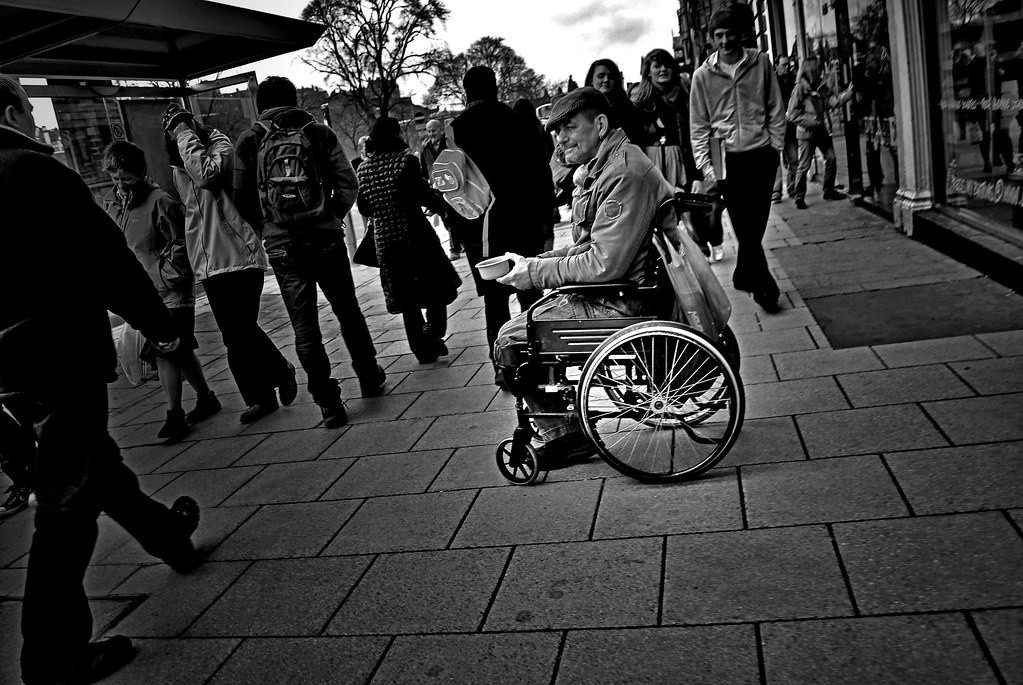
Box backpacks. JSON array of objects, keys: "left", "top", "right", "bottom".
[
  {"left": 253, "top": 115, "right": 328, "bottom": 231},
  {"left": 431, "top": 123, "right": 496, "bottom": 221}
]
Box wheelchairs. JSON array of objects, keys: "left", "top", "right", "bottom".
[{"left": 495, "top": 190, "right": 747, "bottom": 486}]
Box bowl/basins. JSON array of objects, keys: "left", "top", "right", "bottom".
[{"left": 475, "top": 254, "right": 510, "bottom": 281}]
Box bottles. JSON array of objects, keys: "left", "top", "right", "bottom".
[{"left": 675, "top": 264, "right": 715, "bottom": 333}]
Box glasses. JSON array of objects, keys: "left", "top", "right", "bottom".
[{"left": 162, "top": 106, "right": 179, "bottom": 128}]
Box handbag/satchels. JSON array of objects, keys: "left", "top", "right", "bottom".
[
  {"left": 117, "top": 314, "right": 160, "bottom": 386},
  {"left": 651, "top": 223, "right": 732, "bottom": 342}
]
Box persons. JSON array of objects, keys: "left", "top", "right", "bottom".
[
  {"left": 0, "top": 76, "right": 200, "bottom": 685},
  {"left": 557, "top": 50, "right": 724, "bottom": 264},
  {"left": 952, "top": 41, "right": 1023, "bottom": 175},
  {"left": 857, "top": 72, "right": 899, "bottom": 196},
  {"left": 231, "top": 77, "right": 386, "bottom": 429},
  {"left": 162, "top": 103, "right": 297, "bottom": 423},
  {"left": 421, "top": 120, "right": 466, "bottom": 261},
  {"left": 771, "top": 55, "right": 853, "bottom": 210},
  {"left": 357, "top": 117, "right": 462, "bottom": 365},
  {"left": 436, "top": 66, "right": 555, "bottom": 392},
  {"left": 351, "top": 136, "right": 369, "bottom": 231},
  {"left": 103, "top": 142, "right": 221, "bottom": 440},
  {"left": 689, "top": 11, "right": 786, "bottom": 316},
  {"left": 513, "top": 98, "right": 562, "bottom": 253},
  {"left": 492, "top": 86, "right": 680, "bottom": 471}
]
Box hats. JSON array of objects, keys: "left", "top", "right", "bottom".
[
  {"left": 544, "top": 88, "right": 607, "bottom": 132},
  {"left": 162, "top": 103, "right": 194, "bottom": 133}
]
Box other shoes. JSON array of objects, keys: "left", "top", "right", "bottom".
[
  {"left": 322, "top": 402, "right": 348, "bottom": 428},
  {"left": 25, "top": 633, "right": 133, "bottom": 685},
  {"left": 239, "top": 398, "right": 279, "bottom": 424},
  {"left": 157, "top": 407, "right": 187, "bottom": 440},
  {"left": 170, "top": 496, "right": 200, "bottom": 575},
  {"left": 277, "top": 360, "right": 297, "bottom": 406},
  {"left": 824, "top": 190, "right": 847, "bottom": 200},
  {"left": 424, "top": 324, "right": 449, "bottom": 356},
  {"left": 712, "top": 243, "right": 724, "bottom": 261},
  {"left": 795, "top": 199, "right": 807, "bottom": 209},
  {"left": 449, "top": 251, "right": 458, "bottom": 260},
  {"left": 361, "top": 363, "right": 386, "bottom": 397},
  {"left": 526, "top": 427, "right": 607, "bottom": 470},
  {"left": 186, "top": 390, "right": 222, "bottom": 425},
  {"left": 756, "top": 298, "right": 777, "bottom": 315}
]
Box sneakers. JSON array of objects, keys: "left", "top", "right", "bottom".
[{"left": 1, "top": 479, "right": 35, "bottom": 514}]
